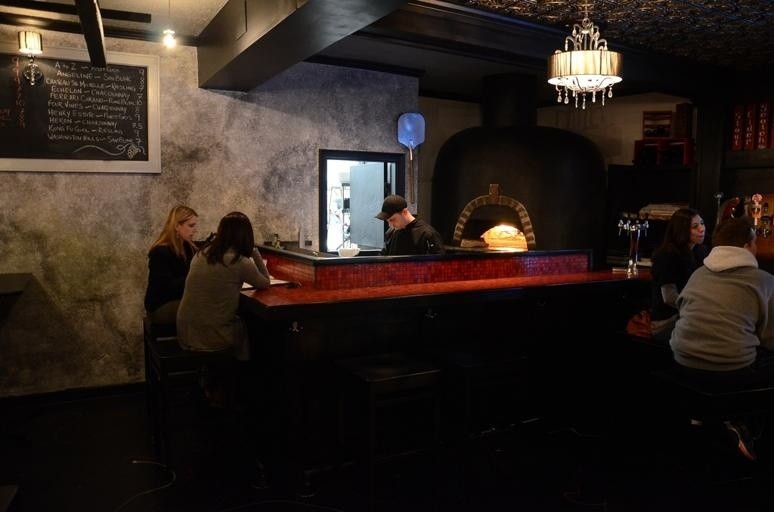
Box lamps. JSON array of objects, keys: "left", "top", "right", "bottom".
[
  {"left": 18, "top": 31, "right": 43, "bottom": 85},
  {"left": 397, "top": 112, "right": 425, "bottom": 162},
  {"left": 547, "top": 0, "right": 624, "bottom": 110},
  {"left": 163, "top": 0, "right": 176, "bottom": 48}
]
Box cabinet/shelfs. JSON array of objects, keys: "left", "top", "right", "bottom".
[
  {"left": 632, "top": 108, "right": 691, "bottom": 164},
  {"left": 605, "top": 163, "right": 689, "bottom": 238}
]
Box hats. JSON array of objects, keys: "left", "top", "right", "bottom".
[{"left": 375, "top": 195, "right": 407, "bottom": 220}]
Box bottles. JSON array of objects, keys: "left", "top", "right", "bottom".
[
  {"left": 272, "top": 234, "right": 280, "bottom": 249},
  {"left": 628, "top": 260, "right": 638, "bottom": 279},
  {"left": 759, "top": 203, "right": 771, "bottom": 239},
  {"left": 631, "top": 125, "right": 682, "bottom": 168},
  {"left": 752, "top": 200, "right": 762, "bottom": 219}
]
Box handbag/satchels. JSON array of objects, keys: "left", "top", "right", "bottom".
[{"left": 627, "top": 309, "right": 651, "bottom": 338}]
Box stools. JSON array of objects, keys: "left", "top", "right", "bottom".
[
  {"left": 431, "top": 353, "right": 528, "bottom": 505},
  {"left": 332, "top": 359, "right": 441, "bottom": 511},
  {"left": 652, "top": 363, "right": 774, "bottom": 510},
  {"left": 148, "top": 339, "right": 243, "bottom": 473},
  {"left": 616, "top": 331, "right": 672, "bottom": 481},
  {"left": 144, "top": 322, "right": 182, "bottom": 434}
]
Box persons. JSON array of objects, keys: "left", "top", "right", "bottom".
[
  {"left": 375, "top": 194, "right": 443, "bottom": 256},
  {"left": 667, "top": 217, "right": 774, "bottom": 465},
  {"left": 144, "top": 207, "right": 195, "bottom": 327},
  {"left": 650, "top": 207, "right": 707, "bottom": 348},
  {"left": 176, "top": 211, "right": 271, "bottom": 410}
]
